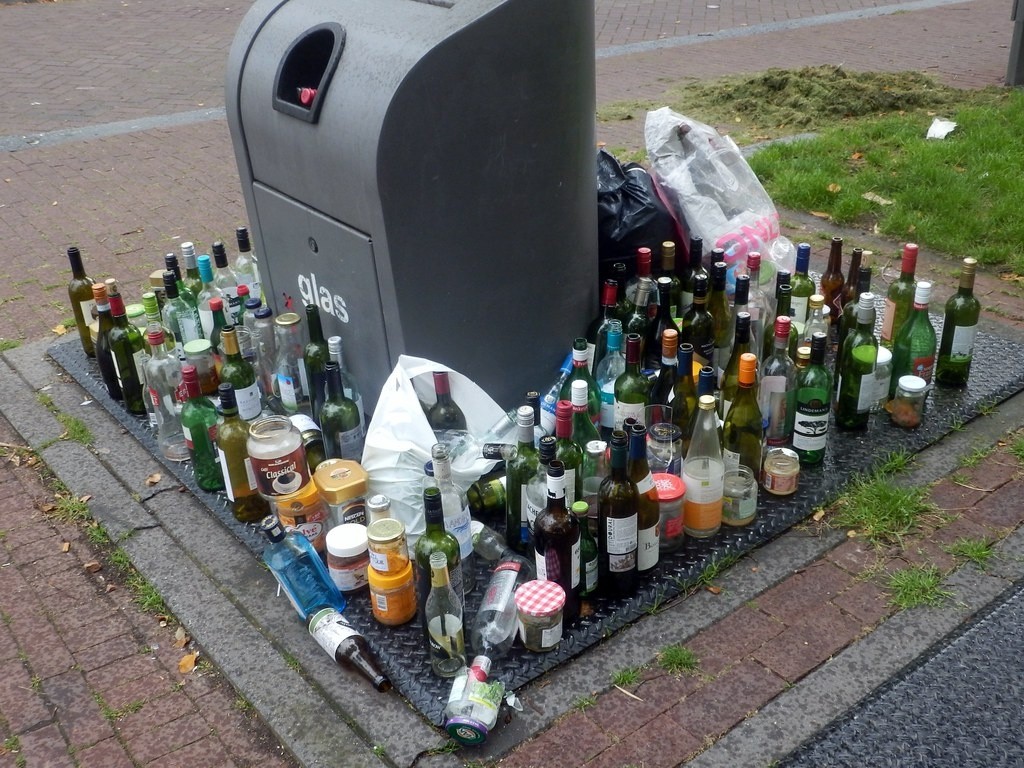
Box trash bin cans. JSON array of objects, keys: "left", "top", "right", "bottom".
[{"left": 222, "top": 1, "right": 599, "bottom": 421}]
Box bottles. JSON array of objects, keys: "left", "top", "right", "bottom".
[
  {"left": 301, "top": 86, "right": 318, "bottom": 108},
  {"left": 62, "top": 228, "right": 981, "bottom": 746}
]
